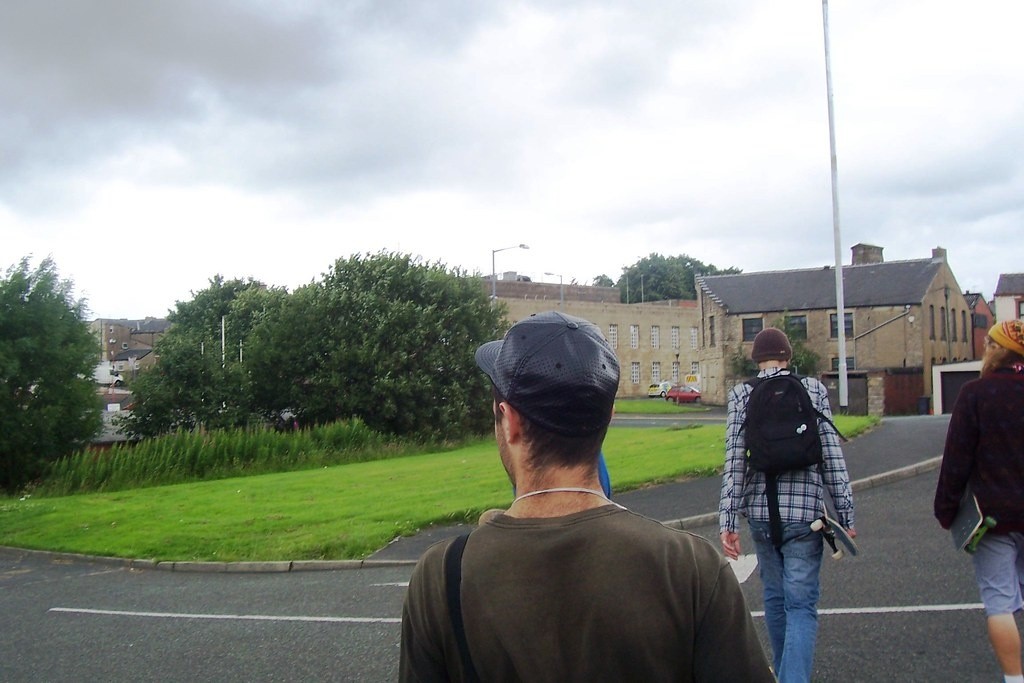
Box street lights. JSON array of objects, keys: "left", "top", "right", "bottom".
[
  {"left": 544, "top": 271, "right": 564, "bottom": 312},
  {"left": 491, "top": 244, "right": 530, "bottom": 303}
]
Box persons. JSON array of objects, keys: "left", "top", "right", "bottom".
[
  {"left": 932, "top": 318, "right": 1024, "bottom": 683},
  {"left": 719, "top": 327, "right": 857, "bottom": 682},
  {"left": 397, "top": 309, "right": 784, "bottom": 683}
]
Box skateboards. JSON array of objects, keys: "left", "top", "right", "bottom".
[
  {"left": 808, "top": 501, "right": 860, "bottom": 561},
  {"left": 949, "top": 489, "right": 998, "bottom": 556}
]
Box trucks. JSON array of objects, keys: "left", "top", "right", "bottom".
[{"left": 78, "top": 364, "right": 125, "bottom": 386}]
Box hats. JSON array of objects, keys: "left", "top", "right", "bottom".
[
  {"left": 988, "top": 319, "right": 1024, "bottom": 355},
  {"left": 752, "top": 329, "right": 791, "bottom": 362},
  {"left": 475, "top": 311, "right": 620, "bottom": 436}
]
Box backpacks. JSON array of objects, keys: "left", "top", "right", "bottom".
[{"left": 739, "top": 373, "right": 847, "bottom": 568}]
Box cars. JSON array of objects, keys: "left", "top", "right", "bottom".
[
  {"left": 665, "top": 385, "right": 702, "bottom": 403},
  {"left": 649, "top": 382, "right": 677, "bottom": 398}
]
